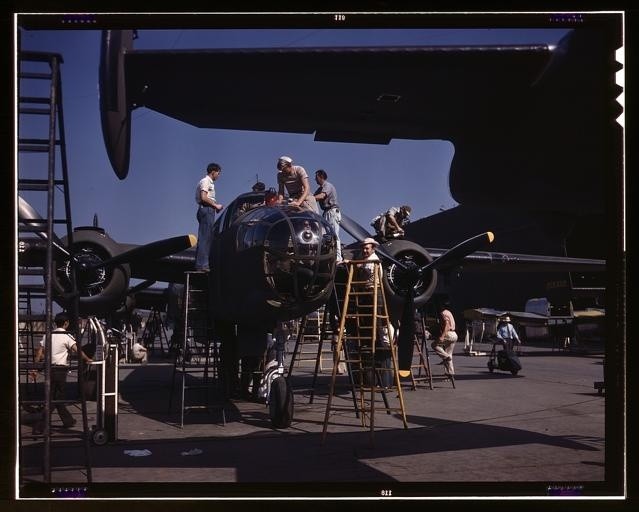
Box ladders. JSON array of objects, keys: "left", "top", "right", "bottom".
[
  {"left": 410, "top": 303, "right": 456, "bottom": 389},
  {"left": 140, "top": 306, "right": 171, "bottom": 355},
  {"left": 17, "top": 47, "right": 93, "bottom": 482},
  {"left": 168, "top": 270, "right": 228, "bottom": 429},
  {"left": 287, "top": 259, "right": 407, "bottom": 449}
]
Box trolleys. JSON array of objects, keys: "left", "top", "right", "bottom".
[{"left": 84, "top": 343, "right": 120, "bottom": 447}]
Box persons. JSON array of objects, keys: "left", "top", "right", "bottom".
[
  {"left": 337, "top": 235, "right": 390, "bottom": 347},
  {"left": 131, "top": 337, "right": 148, "bottom": 363},
  {"left": 286, "top": 217, "right": 332, "bottom": 294},
  {"left": 28, "top": 311, "right": 95, "bottom": 429},
  {"left": 486, "top": 315, "right": 523, "bottom": 377},
  {"left": 430, "top": 300, "right": 458, "bottom": 383},
  {"left": 194, "top": 162, "right": 224, "bottom": 273},
  {"left": 329, "top": 328, "right": 347, "bottom": 375},
  {"left": 312, "top": 168, "right": 344, "bottom": 267},
  {"left": 368, "top": 204, "right": 412, "bottom": 245},
  {"left": 276, "top": 155, "right": 318, "bottom": 216},
  {"left": 370, "top": 315, "right": 397, "bottom": 387}
]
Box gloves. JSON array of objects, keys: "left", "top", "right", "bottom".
[{"left": 336, "top": 258, "right": 353, "bottom": 268}]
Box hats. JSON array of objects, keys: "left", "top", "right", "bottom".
[
  {"left": 359, "top": 238, "right": 380, "bottom": 248},
  {"left": 404, "top": 208, "right": 411, "bottom": 218},
  {"left": 276, "top": 156, "right": 293, "bottom": 171},
  {"left": 502, "top": 316, "right": 512, "bottom": 323}
]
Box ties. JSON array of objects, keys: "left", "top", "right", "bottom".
[{"left": 506, "top": 325, "right": 511, "bottom": 338}]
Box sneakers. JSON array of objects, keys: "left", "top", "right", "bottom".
[
  {"left": 195, "top": 267, "right": 214, "bottom": 274},
  {"left": 439, "top": 356, "right": 452, "bottom": 366}
]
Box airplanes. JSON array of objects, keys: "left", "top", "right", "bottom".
[{"left": 17, "top": 187, "right": 607, "bottom": 428}]
